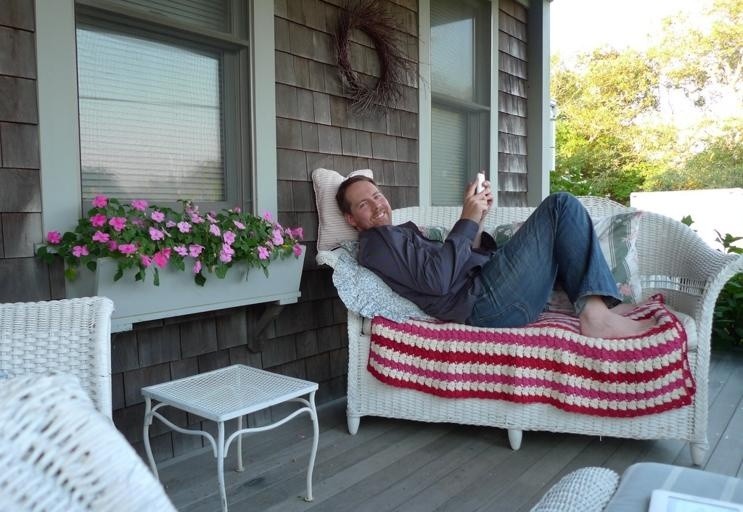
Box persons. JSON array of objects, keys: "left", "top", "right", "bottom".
[{"left": 336, "top": 175, "right": 656, "bottom": 339}]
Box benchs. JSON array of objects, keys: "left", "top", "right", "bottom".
[{"left": 316, "top": 205, "right": 743, "bottom": 467}]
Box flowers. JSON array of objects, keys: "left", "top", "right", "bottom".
[{"left": 33, "top": 193, "right": 305, "bottom": 288}]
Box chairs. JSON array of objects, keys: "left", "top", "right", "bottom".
[
  {"left": 0, "top": 296, "right": 115, "bottom": 421},
  {"left": 527, "top": 461, "right": 743, "bottom": 512}
]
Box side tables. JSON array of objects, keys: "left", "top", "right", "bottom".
[{"left": 139, "top": 362, "right": 321, "bottom": 512}]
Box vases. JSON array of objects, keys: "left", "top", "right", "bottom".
[{"left": 62, "top": 237, "right": 307, "bottom": 334}]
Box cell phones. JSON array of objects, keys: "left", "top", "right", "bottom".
[{"left": 476, "top": 173, "right": 486, "bottom": 194}]
